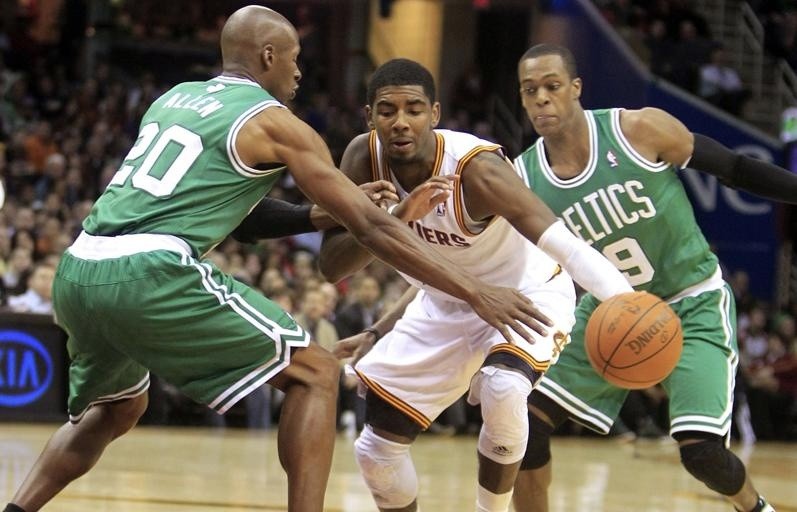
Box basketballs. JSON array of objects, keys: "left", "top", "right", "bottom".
[{"left": 584, "top": 292, "right": 683, "bottom": 389}]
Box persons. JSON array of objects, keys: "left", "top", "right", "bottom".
[
  {"left": 0, "top": 2, "right": 552, "bottom": 510},
  {"left": 327, "top": 40, "right": 797, "bottom": 512},
  {"left": 316, "top": 58, "right": 648, "bottom": 510},
  {"left": 0, "top": 1, "right": 795, "bottom": 443}
]
